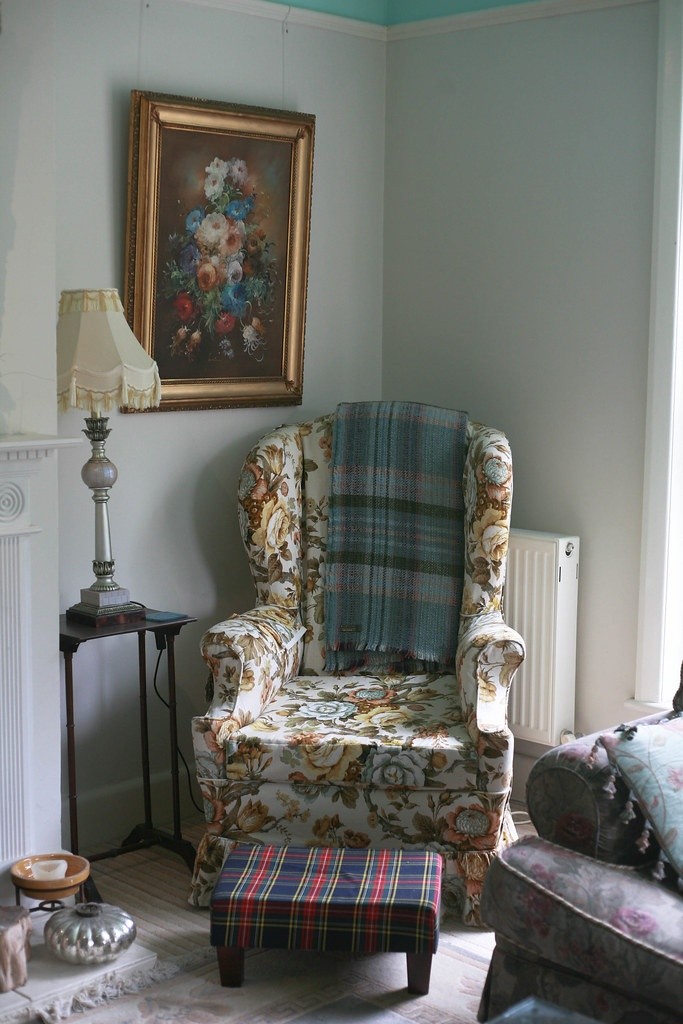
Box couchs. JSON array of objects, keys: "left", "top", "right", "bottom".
[
  {"left": 187, "top": 399, "right": 517, "bottom": 931},
  {"left": 477, "top": 662, "right": 683, "bottom": 1024}
]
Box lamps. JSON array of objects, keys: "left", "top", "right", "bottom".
[{"left": 54, "top": 283, "right": 161, "bottom": 617}]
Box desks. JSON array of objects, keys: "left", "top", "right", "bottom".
[{"left": 52, "top": 608, "right": 196, "bottom": 904}]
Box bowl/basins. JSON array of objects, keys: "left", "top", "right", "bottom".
[{"left": 11, "top": 854, "right": 90, "bottom": 900}]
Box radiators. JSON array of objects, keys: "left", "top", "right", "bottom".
[{"left": 499, "top": 527, "right": 582, "bottom": 753}]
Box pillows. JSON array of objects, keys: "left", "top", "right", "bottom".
[{"left": 587, "top": 713, "right": 683, "bottom": 889}]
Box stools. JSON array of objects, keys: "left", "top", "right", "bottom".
[{"left": 209, "top": 842, "right": 442, "bottom": 1001}]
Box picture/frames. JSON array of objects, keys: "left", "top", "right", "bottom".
[{"left": 129, "top": 87, "right": 314, "bottom": 412}]
